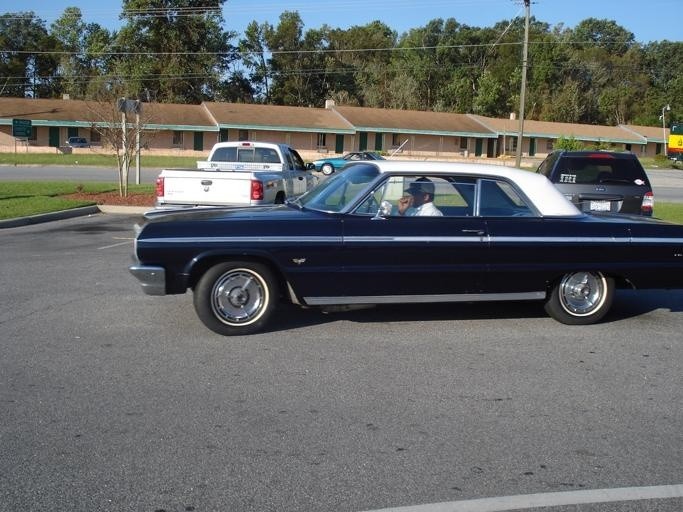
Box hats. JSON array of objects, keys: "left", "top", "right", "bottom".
[{"left": 403, "top": 177, "right": 434, "bottom": 194}]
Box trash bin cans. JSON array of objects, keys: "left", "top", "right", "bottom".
[{"left": 464, "top": 150, "right": 468, "bottom": 158}]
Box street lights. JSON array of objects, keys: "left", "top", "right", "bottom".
[{"left": 660, "top": 104, "right": 670, "bottom": 156}]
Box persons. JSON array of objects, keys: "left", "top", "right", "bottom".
[{"left": 395, "top": 177, "right": 444, "bottom": 216}]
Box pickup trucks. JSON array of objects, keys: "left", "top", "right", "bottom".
[{"left": 157, "top": 139, "right": 320, "bottom": 206}]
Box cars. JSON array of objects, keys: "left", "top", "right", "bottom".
[
  {"left": 65, "top": 137, "right": 91, "bottom": 148},
  {"left": 312, "top": 152, "right": 387, "bottom": 175},
  {"left": 131, "top": 159, "right": 683, "bottom": 337},
  {"left": 536, "top": 149, "right": 654, "bottom": 217}
]
